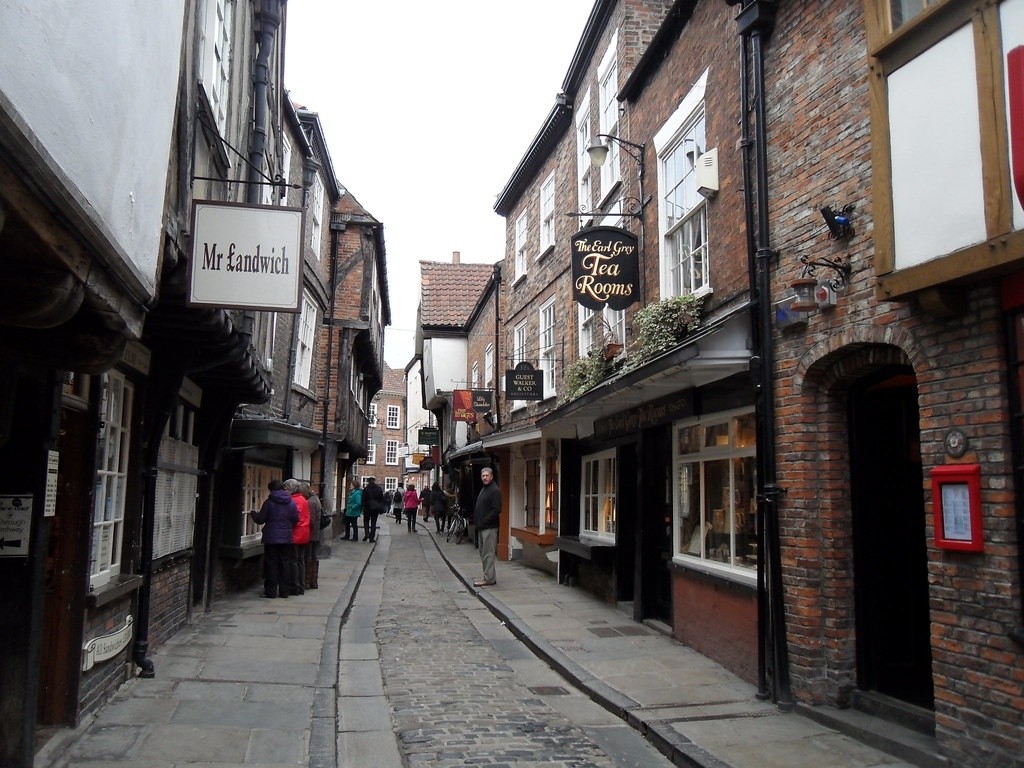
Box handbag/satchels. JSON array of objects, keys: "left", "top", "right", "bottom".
[{"left": 320, "top": 507, "right": 330, "bottom": 530}]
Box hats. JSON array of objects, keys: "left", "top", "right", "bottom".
[{"left": 367, "top": 477, "right": 375, "bottom": 482}]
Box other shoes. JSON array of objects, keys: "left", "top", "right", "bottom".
[
  {"left": 363, "top": 535, "right": 370, "bottom": 541},
  {"left": 436, "top": 529, "right": 440, "bottom": 534},
  {"left": 440, "top": 527, "right": 444, "bottom": 532},
  {"left": 474, "top": 578, "right": 496, "bottom": 587},
  {"left": 260, "top": 593, "right": 277, "bottom": 597},
  {"left": 408, "top": 528, "right": 411, "bottom": 533},
  {"left": 305, "top": 582, "right": 318, "bottom": 590},
  {"left": 423, "top": 518, "right": 428, "bottom": 522},
  {"left": 350, "top": 537, "right": 358, "bottom": 541},
  {"left": 412, "top": 528, "right": 417, "bottom": 532},
  {"left": 277, "top": 593, "right": 288, "bottom": 598},
  {"left": 369, "top": 538, "right": 376, "bottom": 543},
  {"left": 288, "top": 590, "right": 305, "bottom": 596},
  {"left": 340, "top": 535, "right": 350, "bottom": 540}
]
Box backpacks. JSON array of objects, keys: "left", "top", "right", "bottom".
[{"left": 394, "top": 488, "right": 402, "bottom": 503}]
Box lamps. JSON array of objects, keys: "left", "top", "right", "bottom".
[{"left": 790, "top": 254, "right": 851, "bottom": 314}]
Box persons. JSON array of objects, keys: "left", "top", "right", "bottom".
[
  {"left": 299, "top": 482, "right": 324, "bottom": 589},
  {"left": 443, "top": 483, "right": 456, "bottom": 532},
  {"left": 418, "top": 484, "right": 432, "bottom": 523},
  {"left": 362, "top": 477, "right": 384, "bottom": 543},
  {"left": 341, "top": 480, "right": 363, "bottom": 542},
  {"left": 403, "top": 483, "right": 424, "bottom": 534},
  {"left": 283, "top": 478, "right": 311, "bottom": 595},
  {"left": 251, "top": 480, "right": 298, "bottom": 598},
  {"left": 392, "top": 485, "right": 406, "bottom": 524},
  {"left": 432, "top": 482, "right": 446, "bottom": 535},
  {"left": 473, "top": 467, "right": 503, "bottom": 586}
]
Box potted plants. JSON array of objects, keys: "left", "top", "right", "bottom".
[
  {"left": 620, "top": 294, "right": 703, "bottom": 375},
  {"left": 552, "top": 312, "right": 624, "bottom": 409}
]
